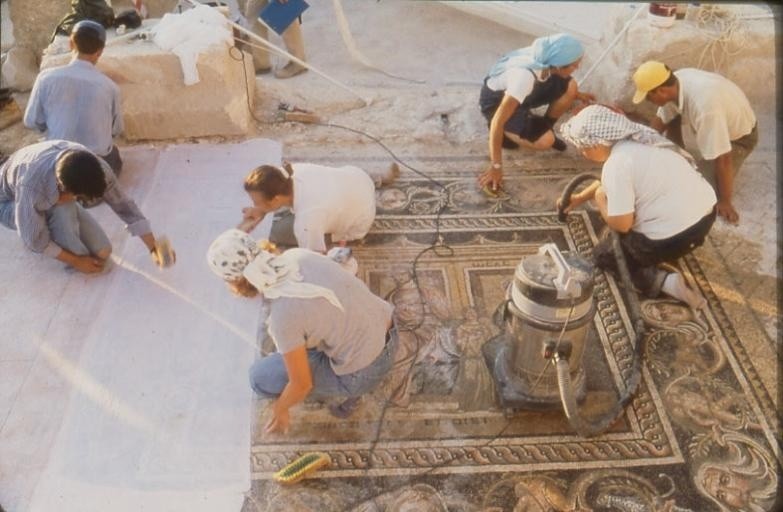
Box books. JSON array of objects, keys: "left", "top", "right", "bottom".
[{"left": 257, "top": 0, "right": 310, "bottom": 39}]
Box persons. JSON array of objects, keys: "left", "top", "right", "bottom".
[
  {"left": 443, "top": 304, "right": 502, "bottom": 411},
  {"left": 234, "top": 161, "right": 406, "bottom": 255},
  {"left": 471, "top": 33, "right": 597, "bottom": 196},
  {"left": 632, "top": 59, "right": 760, "bottom": 227},
  {"left": 22, "top": 18, "right": 125, "bottom": 178},
  {"left": 663, "top": 387, "right": 761, "bottom": 435},
  {"left": 237, "top": 1, "right": 314, "bottom": 80},
  {"left": 555, "top": 105, "right": 716, "bottom": 316},
  {"left": 694, "top": 445, "right": 782, "bottom": 512},
  {"left": 378, "top": 261, "right": 458, "bottom": 410},
  {"left": 206, "top": 225, "right": 403, "bottom": 438},
  {"left": 640, "top": 296, "right": 695, "bottom": 333},
  {"left": 0, "top": 140, "right": 176, "bottom": 273}
]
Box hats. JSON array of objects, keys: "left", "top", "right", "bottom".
[
  {"left": 72, "top": 19, "right": 106, "bottom": 43},
  {"left": 631, "top": 60, "right": 672, "bottom": 104}
]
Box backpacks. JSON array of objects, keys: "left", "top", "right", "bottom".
[{"left": 50, "top": 0, "right": 114, "bottom": 43}]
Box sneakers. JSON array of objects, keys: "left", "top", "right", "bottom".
[
  {"left": 274, "top": 62, "right": 307, "bottom": 79},
  {"left": 668, "top": 273, "right": 707, "bottom": 310},
  {"left": 253, "top": 63, "right": 272, "bottom": 74}
]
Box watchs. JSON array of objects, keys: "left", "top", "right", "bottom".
[{"left": 489, "top": 161, "right": 504, "bottom": 171}]
